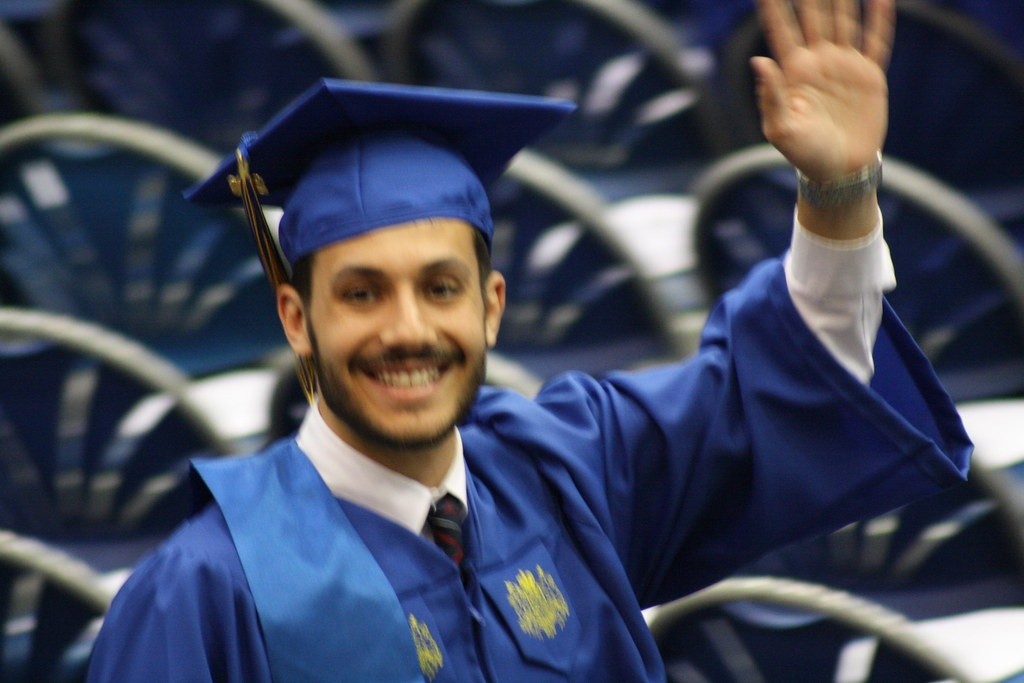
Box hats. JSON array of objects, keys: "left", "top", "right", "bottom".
[{"left": 183, "top": 76, "right": 579, "bottom": 405}]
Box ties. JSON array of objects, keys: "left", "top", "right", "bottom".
[{"left": 426, "top": 493, "right": 468, "bottom": 567}]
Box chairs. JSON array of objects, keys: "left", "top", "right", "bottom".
[{"left": 1, "top": 0, "right": 1024, "bottom": 683}]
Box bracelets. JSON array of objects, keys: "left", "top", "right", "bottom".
[{"left": 796, "top": 163, "right": 882, "bottom": 206}]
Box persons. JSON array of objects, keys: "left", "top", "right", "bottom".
[{"left": 88, "top": 0, "right": 973, "bottom": 683}]
